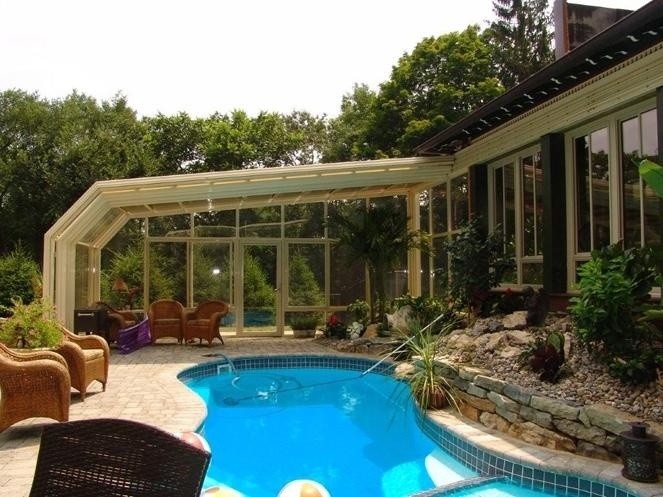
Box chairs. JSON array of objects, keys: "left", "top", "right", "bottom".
[
  {"left": 0, "top": 318, "right": 212, "bottom": 496},
  {"left": 72, "top": 297, "right": 230, "bottom": 348}
]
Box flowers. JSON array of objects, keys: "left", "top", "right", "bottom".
[{"left": 327, "top": 312, "right": 338, "bottom": 327}]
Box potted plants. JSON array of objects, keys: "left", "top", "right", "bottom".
[
  {"left": 376, "top": 310, "right": 464, "bottom": 424},
  {"left": 288, "top": 314, "right": 320, "bottom": 336}
]
